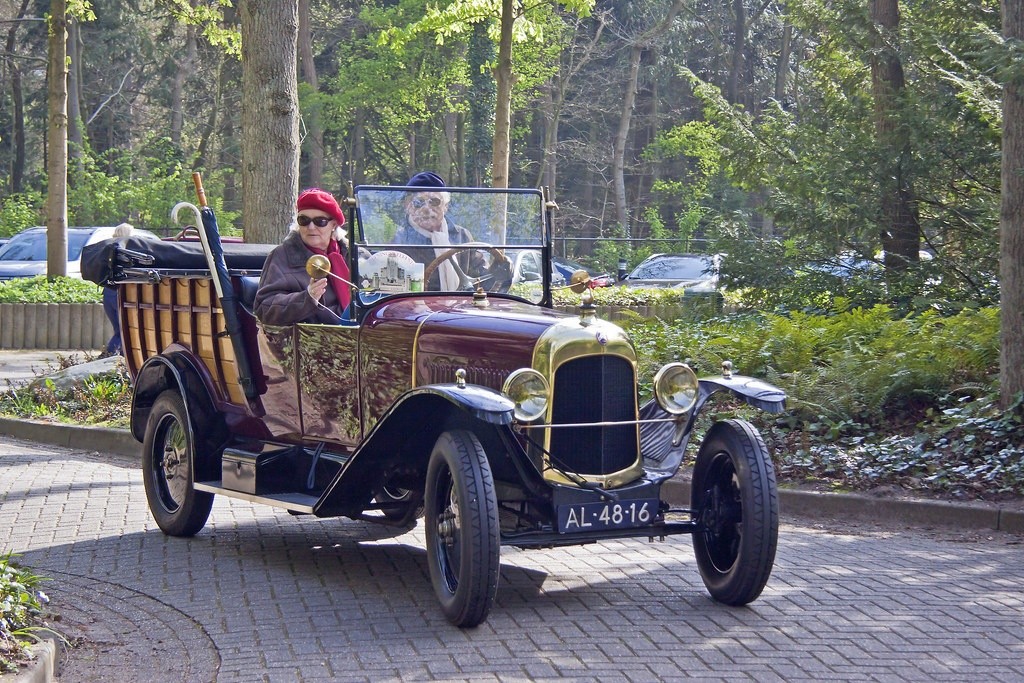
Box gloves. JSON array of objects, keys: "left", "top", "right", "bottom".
[{"left": 488, "top": 255, "right": 514, "bottom": 282}]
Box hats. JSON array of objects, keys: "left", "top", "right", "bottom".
[
  {"left": 297, "top": 188, "right": 345, "bottom": 227},
  {"left": 400, "top": 171, "right": 446, "bottom": 201}
]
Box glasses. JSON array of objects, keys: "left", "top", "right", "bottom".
[
  {"left": 297, "top": 215, "right": 333, "bottom": 227},
  {"left": 407, "top": 198, "right": 444, "bottom": 212}
]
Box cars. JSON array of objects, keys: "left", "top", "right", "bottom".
[
  {"left": 0, "top": 224, "right": 163, "bottom": 285},
  {"left": 612, "top": 251, "right": 749, "bottom": 304},
  {"left": 500, "top": 250, "right": 616, "bottom": 293}
]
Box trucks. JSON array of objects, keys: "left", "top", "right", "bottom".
[{"left": 109, "top": 184, "right": 792, "bottom": 631}]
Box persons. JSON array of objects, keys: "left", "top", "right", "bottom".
[
  {"left": 252, "top": 188, "right": 373, "bottom": 326},
  {"left": 384, "top": 172, "right": 514, "bottom": 298}
]
